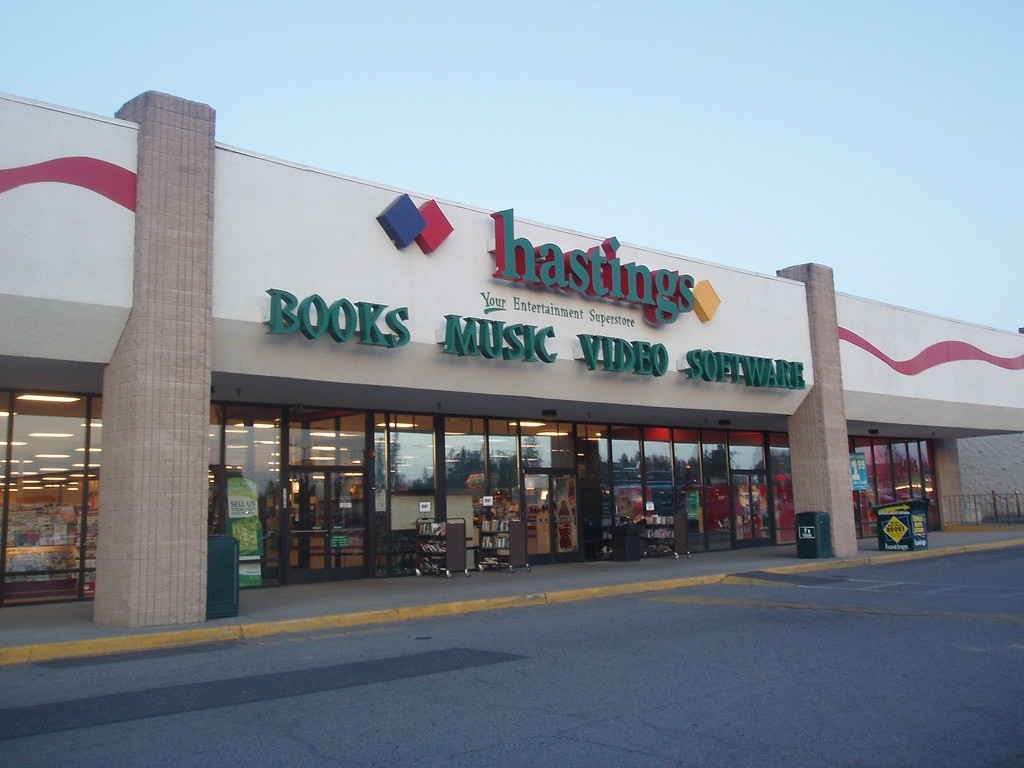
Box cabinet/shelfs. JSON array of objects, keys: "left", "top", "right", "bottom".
[
  {"left": 376, "top": 518, "right": 532, "bottom": 578},
  {"left": 614, "top": 517, "right": 674, "bottom": 560}
]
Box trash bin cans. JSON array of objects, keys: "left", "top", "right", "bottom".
[
  {"left": 796, "top": 511, "right": 833, "bottom": 559},
  {"left": 207, "top": 535, "right": 239, "bottom": 620}
]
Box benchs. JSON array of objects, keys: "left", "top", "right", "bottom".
[{"left": 293, "top": 529, "right": 365, "bottom": 556}]
